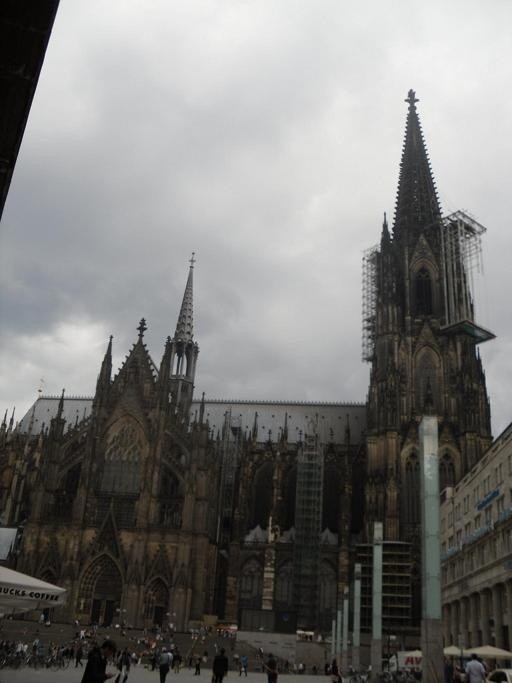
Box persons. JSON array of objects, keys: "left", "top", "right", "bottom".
[
  {"left": 443, "top": 652, "right": 504, "bottom": 683},
  {"left": 1, "top": 610, "right": 424, "bottom": 682}
]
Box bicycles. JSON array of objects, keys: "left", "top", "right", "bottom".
[{"left": 0, "top": 646, "right": 70, "bottom": 671}]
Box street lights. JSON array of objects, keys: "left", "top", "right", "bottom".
[
  {"left": 371, "top": 519, "right": 385, "bottom": 682},
  {"left": 330, "top": 586, "right": 349, "bottom": 674},
  {"left": 353, "top": 562, "right": 362, "bottom": 671},
  {"left": 418, "top": 414, "right": 445, "bottom": 683}
]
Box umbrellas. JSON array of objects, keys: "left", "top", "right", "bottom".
[
  {"left": 460, "top": 645, "right": 512, "bottom": 672},
  {"left": 443, "top": 643, "right": 463, "bottom": 680}
]
